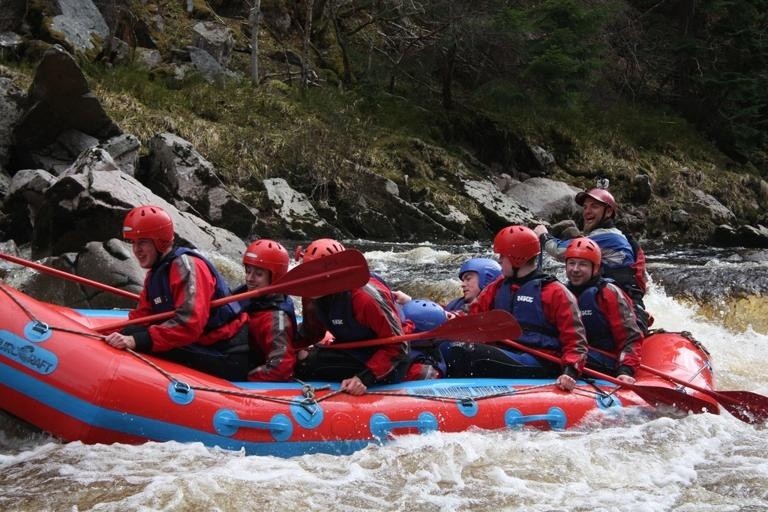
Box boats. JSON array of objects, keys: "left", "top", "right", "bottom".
[{"left": 0, "top": 280, "right": 721, "bottom": 457}]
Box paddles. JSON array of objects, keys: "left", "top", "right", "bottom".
[
  {"left": 312, "top": 310, "right": 522, "bottom": 349},
  {"left": 501, "top": 339, "right": 720, "bottom": 414},
  {"left": 588, "top": 347, "right": 767, "bottom": 425},
  {"left": 91, "top": 249, "right": 371, "bottom": 334}
]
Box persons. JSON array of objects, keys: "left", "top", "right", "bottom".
[
  {"left": 99, "top": 206, "right": 246, "bottom": 384},
  {"left": 233, "top": 239, "right": 298, "bottom": 383},
  {"left": 296, "top": 238, "right": 409, "bottom": 397},
  {"left": 392, "top": 189, "right": 649, "bottom": 391}
]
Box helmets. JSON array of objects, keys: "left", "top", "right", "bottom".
[
  {"left": 397, "top": 298, "right": 448, "bottom": 348},
  {"left": 239, "top": 238, "right": 291, "bottom": 287},
  {"left": 564, "top": 236, "right": 602, "bottom": 267},
  {"left": 121, "top": 205, "right": 174, "bottom": 254},
  {"left": 492, "top": 224, "right": 542, "bottom": 270},
  {"left": 457, "top": 258, "right": 503, "bottom": 290},
  {"left": 300, "top": 238, "right": 347, "bottom": 266},
  {"left": 573, "top": 187, "right": 617, "bottom": 212}
]
